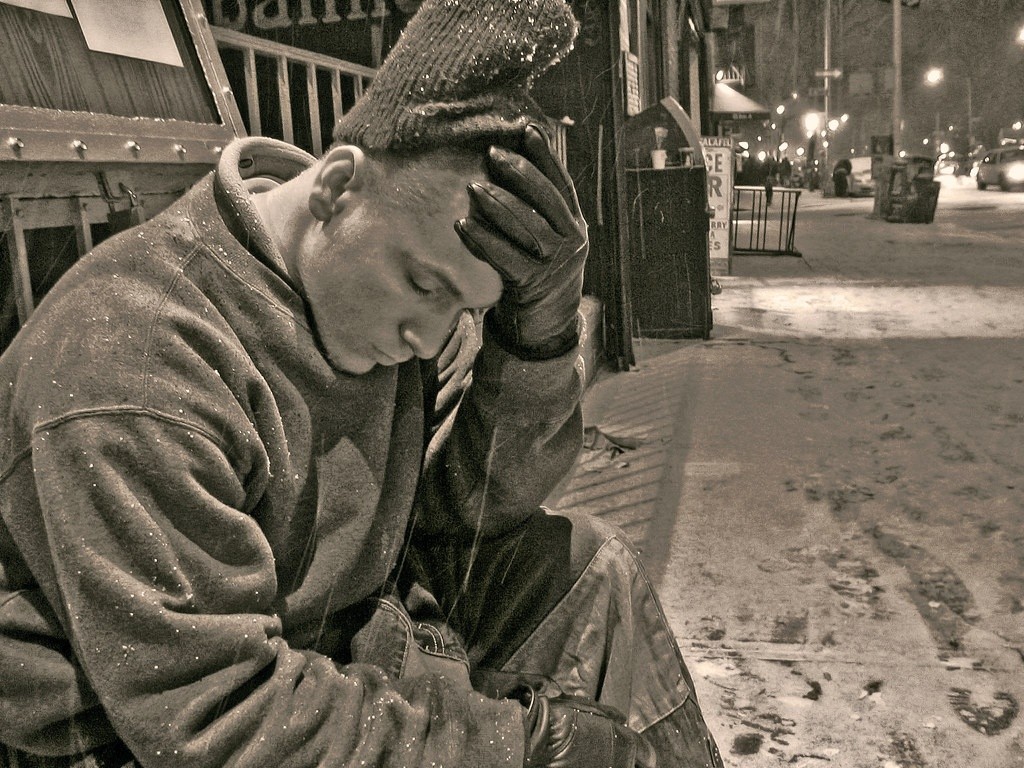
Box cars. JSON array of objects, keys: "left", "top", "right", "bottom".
[
  {"left": 974, "top": 147, "right": 1024, "bottom": 192},
  {"left": 935, "top": 152, "right": 972, "bottom": 178},
  {"left": 832, "top": 157, "right": 875, "bottom": 197}
]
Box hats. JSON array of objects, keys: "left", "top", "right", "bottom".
[{"left": 332, "top": 0, "right": 580, "bottom": 158}]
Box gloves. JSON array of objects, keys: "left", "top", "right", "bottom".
[
  {"left": 506, "top": 682, "right": 656, "bottom": 768},
  {"left": 454, "top": 123, "right": 589, "bottom": 362}
]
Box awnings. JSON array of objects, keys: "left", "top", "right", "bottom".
[{"left": 713, "top": 83, "right": 770, "bottom": 120}]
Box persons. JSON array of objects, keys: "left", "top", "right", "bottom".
[
  {"left": 0, "top": 1, "right": 723, "bottom": 768},
  {"left": 743, "top": 156, "right": 792, "bottom": 187},
  {"left": 833, "top": 159, "right": 851, "bottom": 197}
]
